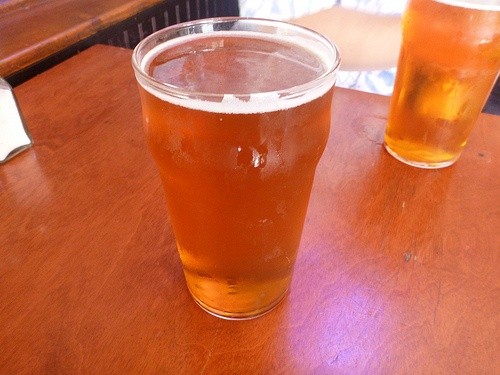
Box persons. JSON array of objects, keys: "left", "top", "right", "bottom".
[{"left": 238, "top": 0, "right": 405, "bottom": 96}]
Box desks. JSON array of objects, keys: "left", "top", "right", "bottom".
[
  {"left": 0, "top": 0, "right": 239, "bottom": 88},
  {"left": 0, "top": 44, "right": 500, "bottom": 375}
]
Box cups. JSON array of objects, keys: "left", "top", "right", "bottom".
[
  {"left": 132, "top": 17, "right": 341, "bottom": 321},
  {"left": 383, "top": 0, "right": 500, "bottom": 168}
]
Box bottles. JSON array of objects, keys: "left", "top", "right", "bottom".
[{"left": 0, "top": 78, "right": 33, "bottom": 164}]
